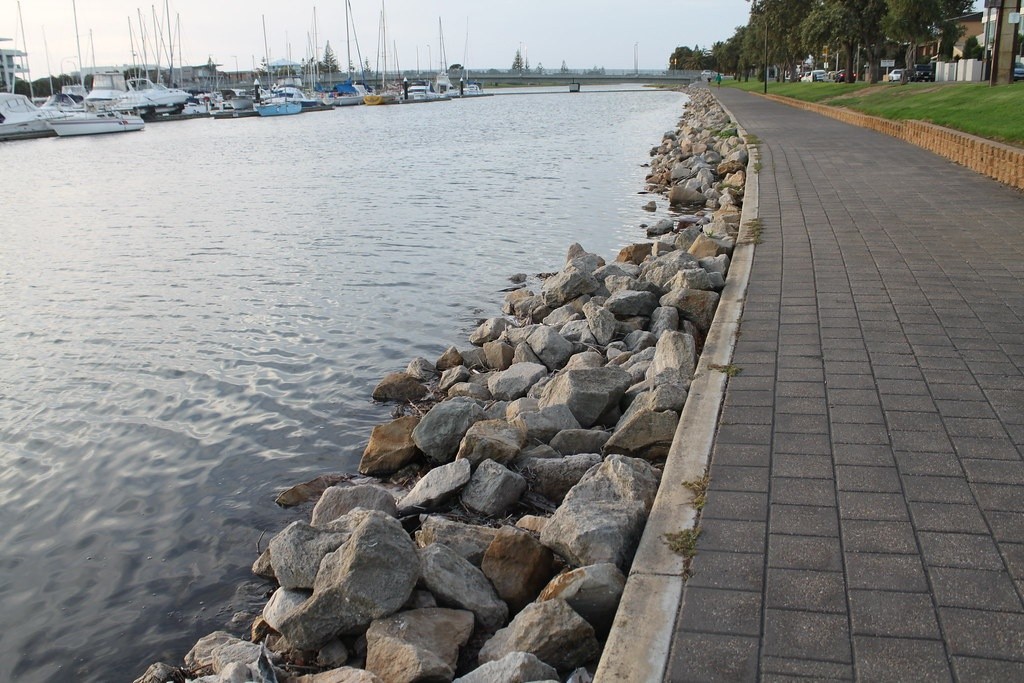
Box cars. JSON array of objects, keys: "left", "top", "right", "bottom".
[{"left": 798, "top": 54, "right": 1024, "bottom": 81}]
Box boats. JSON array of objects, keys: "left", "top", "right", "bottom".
[{"left": 0, "top": 0, "right": 490, "bottom": 140}]
